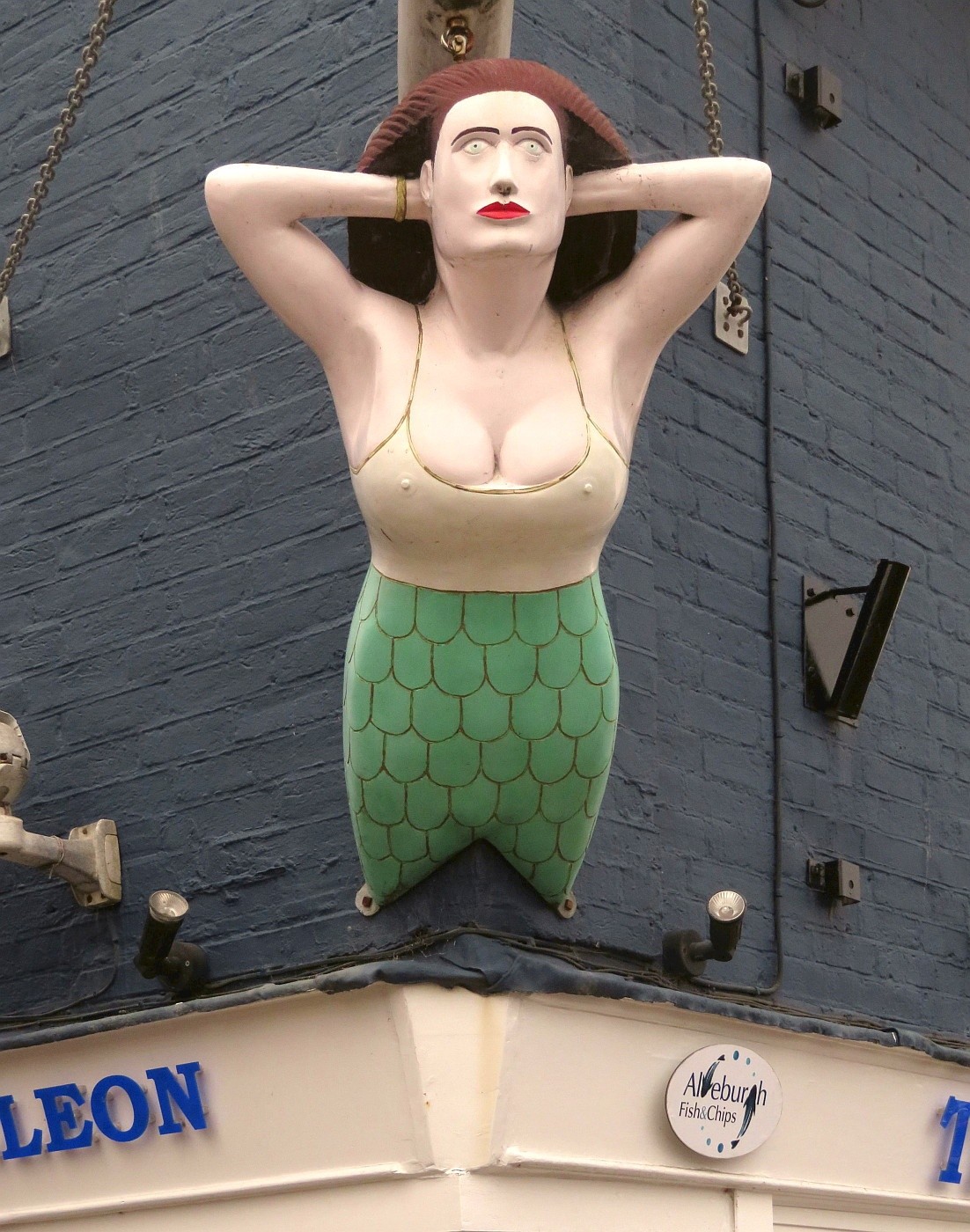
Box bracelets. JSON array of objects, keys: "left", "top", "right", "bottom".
[{"left": 395, "top": 175, "right": 407, "bottom": 222}]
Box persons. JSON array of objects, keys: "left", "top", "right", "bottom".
[{"left": 200, "top": 54, "right": 770, "bottom": 906}]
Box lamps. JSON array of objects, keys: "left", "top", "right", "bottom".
[
  {"left": 133, "top": 889, "right": 210, "bottom": 993},
  {"left": 664, "top": 890, "right": 747, "bottom": 978}
]
